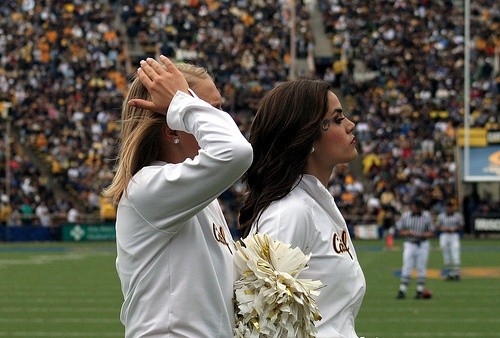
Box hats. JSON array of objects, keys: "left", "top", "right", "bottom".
[{"left": 414, "top": 197, "right": 426, "bottom": 206}]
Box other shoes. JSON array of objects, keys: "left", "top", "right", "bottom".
[
  {"left": 446, "top": 272, "right": 460, "bottom": 281},
  {"left": 399, "top": 290, "right": 404, "bottom": 297},
  {"left": 416, "top": 288, "right": 432, "bottom": 298}
]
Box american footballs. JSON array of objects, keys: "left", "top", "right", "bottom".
[{"left": 422, "top": 291, "right": 432, "bottom": 298}]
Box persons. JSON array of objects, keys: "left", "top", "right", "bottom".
[
  {"left": 396, "top": 199, "right": 434, "bottom": 299},
  {"left": 435, "top": 198, "right": 464, "bottom": 277},
  {"left": 0, "top": 0, "right": 500, "bottom": 240},
  {"left": 99, "top": 56, "right": 253, "bottom": 338},
  {"left": 237, "top": 80, "right": 366, "bottom": 338}
]
